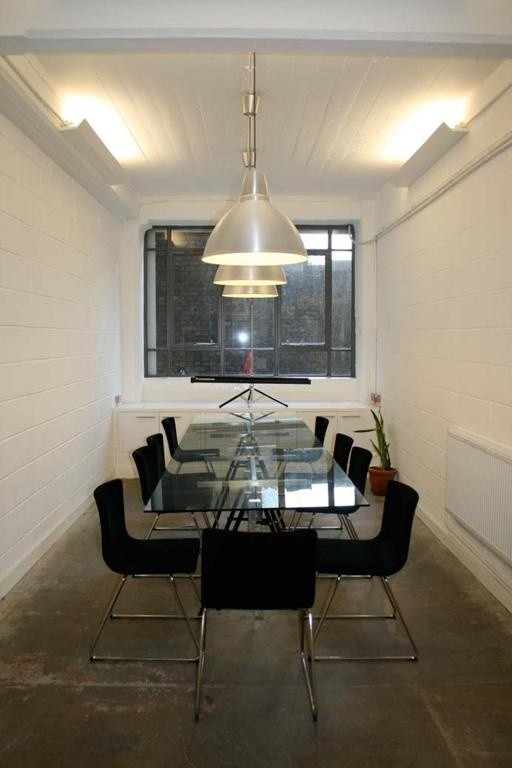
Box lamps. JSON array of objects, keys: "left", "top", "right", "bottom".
[{"left": 199, "top": 52, "right": 308, "bottom": 299}]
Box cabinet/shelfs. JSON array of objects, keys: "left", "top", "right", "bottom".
[
  {"left": 295, "top": 411, "right": 380, "bottom": 478},
  {"left": 117, "top": 411, "right": 200, "bottom": 474}
]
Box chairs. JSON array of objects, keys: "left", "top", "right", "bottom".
[
  {"left": 280, "top": 432, "right": 354, "bottom": 483},
  {"left": 147, "top": 434, "right": 217, "bottom": 481},
  {"left": 86, "top": 478, "right": 203, "bottom": 666},
  {"left": 132, "top": 446, "right": 211, "bottom": 540},
  {"left": 162, "top": 417, "right": 220, "bottom": 473},
  {"left": 307, "top": 478, "right": 420, "bottom": 664},
  {"left": 269, "top": 416, "right": 330, "bottom": 476},
  {"left": 287, "top": 446, "right": 375, "bottom": 539}
]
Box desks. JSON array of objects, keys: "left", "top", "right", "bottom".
[{"left": 142, "top": 411, "right": 369, "bottom": 531}]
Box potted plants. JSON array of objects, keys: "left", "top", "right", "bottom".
[{"left": 352, "top": 409, "right": 397, "bottom": 496}]
